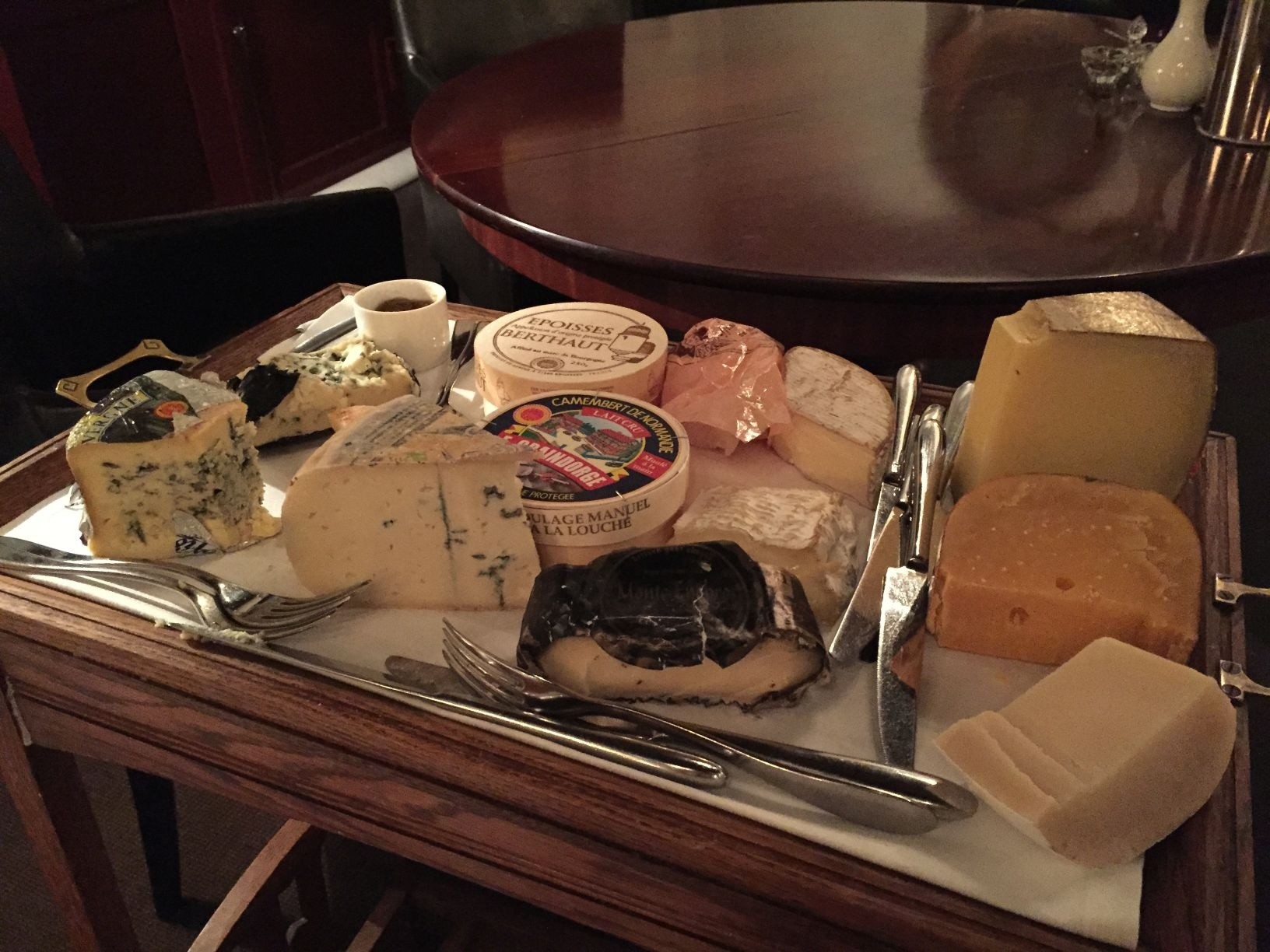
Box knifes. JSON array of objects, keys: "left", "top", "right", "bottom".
[
  {"left": 822, "top": 365, "right": 976, "bottom": 772},
  {"left": 162, "top": 621, "right": 732, "bottom": 790},
  {"left": 384, "top": 656, "right": 748, "bottom": 762}
]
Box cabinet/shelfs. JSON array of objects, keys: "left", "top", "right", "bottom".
[{"left": 0, "top": 278, "right": 1260, "bottom": 950}]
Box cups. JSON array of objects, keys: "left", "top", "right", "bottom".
[{"left": 353, "top": 280, "right": 450, "bottom": 373}]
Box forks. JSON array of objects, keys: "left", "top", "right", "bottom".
[
  {"left": 440, "top": 616, "right": 978, "bottom": 820},
  {"left": 1, "top": 534, "right": 374, "bottom": 639}
]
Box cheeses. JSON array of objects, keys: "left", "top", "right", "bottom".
[{"left": 65, "top": 288, "right": 1242, "bottom": 876}]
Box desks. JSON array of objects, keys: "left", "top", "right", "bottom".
[{"left": 407, "top": 0, "right": 1269, "bottom": 381}]
